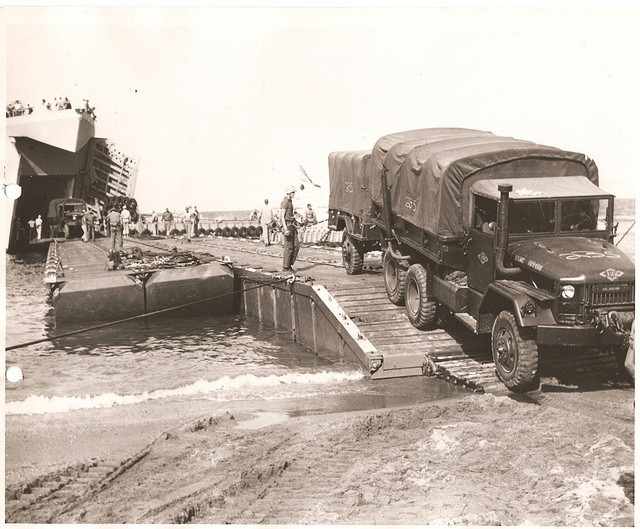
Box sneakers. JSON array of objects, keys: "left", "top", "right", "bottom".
[{"left": 283, "top": 267, "right": 296, "bottom": 274}]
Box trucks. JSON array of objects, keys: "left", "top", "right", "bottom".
[
  {"left": 328, "top": 127, "right": 633, "bottom": 393},
  {"left": 47, "top": 198, "right": 87, "bottom": 239}
]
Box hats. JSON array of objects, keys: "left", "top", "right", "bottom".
[{"left": 285, "top": 185, "right": 296, "bottom": 193}]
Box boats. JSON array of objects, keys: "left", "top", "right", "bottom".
[{"left": 5, "top": 111, "right": 93, "bottom": 251}]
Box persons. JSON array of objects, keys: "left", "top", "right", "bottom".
[
  {"left": 88, "top": 211, "right": 97, "bottom": 241},
  {"left": 161, "top": 208, "right": 173, "bottom": 236},
  {"left": 135, "top": 211, "right": 146, "bottom": 239},
  {"left": 150, "top": 211, "right": 158, "bottom": 237},
  {"left": 80, "top": 213, "right": 89, "bottom": 242},
  {"left": 35, "top": 215, "right": 43, "bottom": 240},
  {"left": 6, "top": 96, "right": 71, "bottom": 117},
  {"left": 258, "top": 199, "right": 274, "bottom": 246},
  {"left": 302, "top": 204, "right": 318, "bottom": 230},
  {"left": 106, "top": 208, "right": 123, "bottom": 251},
  {"left": 121, "top": 205, "right": 131, "bottom": 238},
  {"left": 280, "top": 187, "right": 300, "bottom": 274},
  {"left": 27, "top": 218, "right": 36, "bottom": 241},
  {"left": 192, "top": 206, "right": 202, "bottom": 236},
  {"left": 182, "top": 207, "right": 193, "bottom": 240}
]
